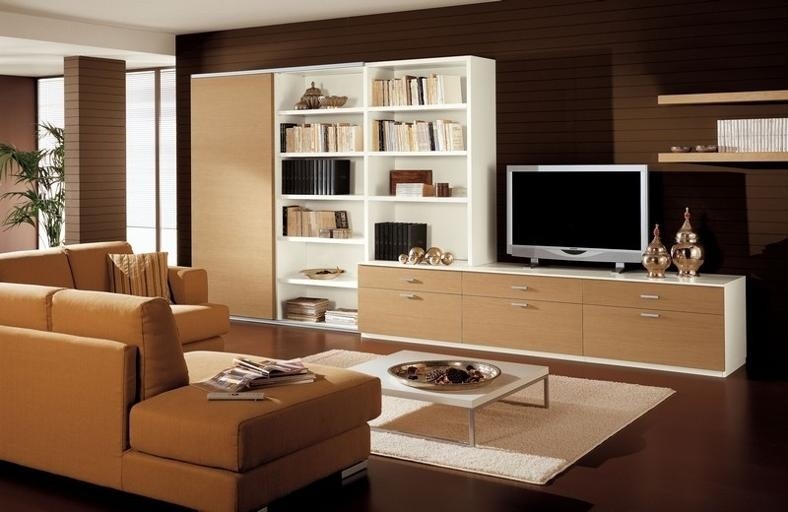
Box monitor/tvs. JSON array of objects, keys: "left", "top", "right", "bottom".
[{"left": 507, "top": 164, "right": 648, "bottom": 274}]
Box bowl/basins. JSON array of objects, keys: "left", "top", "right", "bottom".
[
  {"left": 318, "top": 95, "right": 348, "bottom": 109},
  {"left": 696, "top": 144, "right": 717, "bottom": 153},
  {"left": 670, "top": 146, "right": 692, "bottom": 152}
]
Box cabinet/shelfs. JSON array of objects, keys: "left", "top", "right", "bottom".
[
  {"left": 656, "top": 87, "right": 788, "bottom": 168},
  {"left": 355, "top": 258, "right": 746, "bottom": 380},
  {"left": 186, "top": 53, "right": 500, "bottom": 332}
]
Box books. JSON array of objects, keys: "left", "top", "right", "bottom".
[
  {"left": 396, "top": 182, "right": 433, "bottom": 197},
  {"left": 202, "top": 357, "right": 317, "bottom": 392},
  {"left": 372, "top": 118, "right": 463, "bottom": 151},
  {"left": 371, "top": 73, "right": 462, "bottom": 106},
  {"left": 283, "top": 297, "right": 358, "bottom": 325},
  {"left": 280, "top": 123, "right": 362, "bottom": 153},
  {"left": 282, "top": 160, "right": 350, "bottom": 195},
  {"left": 283, "top": 206, "right": 352, "bottom": 239},
  {"left": 375, "top": 223, "right": 426, "bottom": 261},
  {"left": 717, "top": 118, "right": 788, "bottom": 152}
]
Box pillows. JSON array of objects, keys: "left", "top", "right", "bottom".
[{"left": 105, "top": 251, "right": 174, "bottom": 305}]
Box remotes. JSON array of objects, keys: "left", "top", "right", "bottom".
[{"left": 207, "top": 392, "right": 264, "bottom": 400}]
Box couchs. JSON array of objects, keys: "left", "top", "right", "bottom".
[
  {"left": 0, "top": 280, "right": 385, "bottom": 512},
  {"left": 0, "top": 237, "right": 233, "bottom": 344}
]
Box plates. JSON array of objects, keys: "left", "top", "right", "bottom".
[{"left": 300, "top": 268, "right": 346, "bottom": 281}]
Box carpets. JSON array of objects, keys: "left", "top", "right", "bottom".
[{"left": 298, "top": 348, "right": 678, "bottom": 486}]
